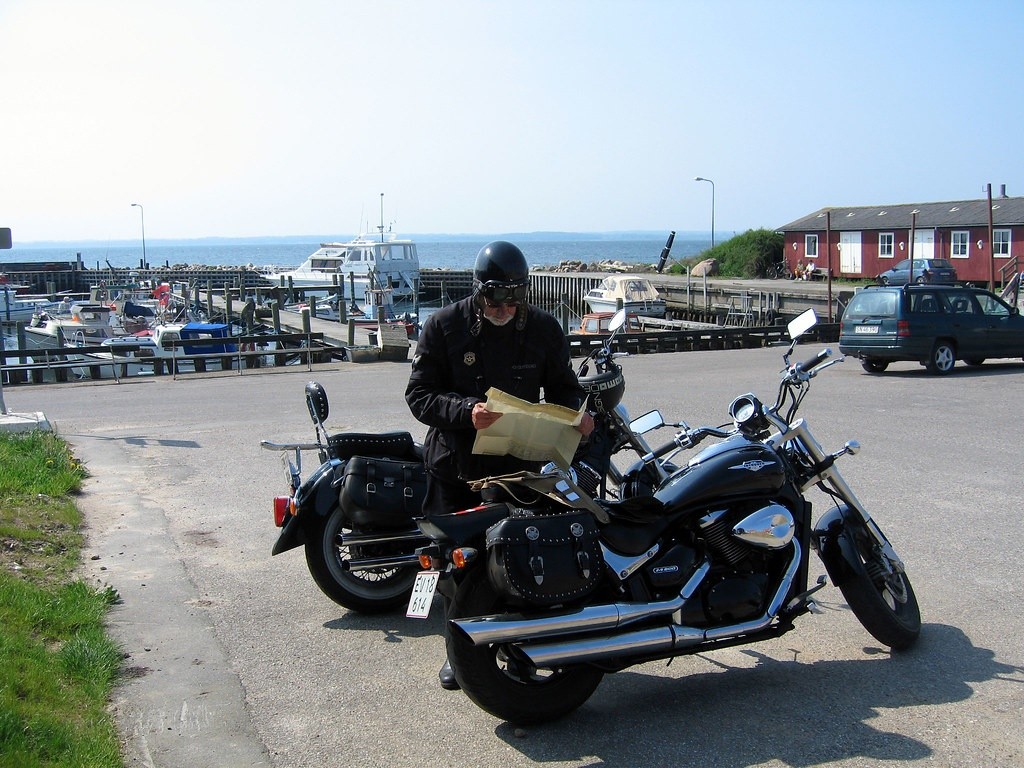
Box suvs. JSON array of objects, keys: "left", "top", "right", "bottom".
[{"left": 839, "top": 282, "right": 1024, "bottom": 377}]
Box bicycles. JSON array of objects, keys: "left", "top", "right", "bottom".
[{"left": 768, "top": 258, "right": 795, "bottom": 281}]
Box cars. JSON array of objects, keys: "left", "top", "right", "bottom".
[{"left": 879, "top": 258, "right": 958, "bottom": 287}]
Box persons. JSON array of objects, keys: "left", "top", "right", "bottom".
[
  {"left": 793, "top": 259, "right": 806, "bottom": 281},
  {"left": 405, "top": 241, "right": 595, "bottom": 689},
  {"left": 1019, "top": 271, "right": 1024, "bottom": 286},
  {"left": 802, "top": 260, "right": 816, "bottom": 281},
  {"left": 57, "top": 297, "right": 71, "bottom": 315}
]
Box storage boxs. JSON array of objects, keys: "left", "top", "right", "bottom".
[{"left": 345, "top": 345, "right": 381, "bottom": 363}]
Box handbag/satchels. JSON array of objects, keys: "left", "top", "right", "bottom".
[{"left": 466, "top": 470, "right": 610, "bottom": 524}]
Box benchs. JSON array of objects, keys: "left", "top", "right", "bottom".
[
  {"left": 0, "top": 345, "right": 142, "bottom": 386},
  {"left": 161, "top": 332, "right": 325, "bottom": 381},
  {"left": 812, "top": 268, "right": 828, "bottom": 283}
]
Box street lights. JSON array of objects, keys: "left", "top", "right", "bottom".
[
  {"left": 131, "top": 203, "right": 146, "bottom": 269},
  {"left": 693, "top": 176, "right": 714, "bottom": 250}
]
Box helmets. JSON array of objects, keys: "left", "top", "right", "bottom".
[{"left": 473, "top": 240, "right": 529, "bottom": 284}]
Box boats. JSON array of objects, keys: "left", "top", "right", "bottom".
[
  {"left": 582, "top": 275, "right": 667, "bottom": 318},
  {"left": 0, "top": 279, "right": 419, "bottom": 378},
  {"left": 260, "top": 192, "right": 427, "bottom": 301},
  {"left": 568, "top": 311, "right": 643, "bottom": 341}
]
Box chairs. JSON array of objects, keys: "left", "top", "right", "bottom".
[
  {"left": 919, "top": 299, "right": 937, "bottom": 313},
  {"left": 951, "top": 299, "right": 968, "bottom": 312}
]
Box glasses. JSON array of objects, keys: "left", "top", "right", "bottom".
[
  {"left": 483, "top": 295, "right": 524, "bottom": 308},
  {"left": 472, "top": 278, "right": 532, "bottom": 301}
]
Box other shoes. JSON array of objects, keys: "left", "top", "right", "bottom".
[{"left": 438, "top": 659, "right": 460, "bottom": 689}]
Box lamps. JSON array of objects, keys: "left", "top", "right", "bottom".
[
  {"left": 977, "top": 240, "right": 983, "bottom": 250},
  {"left": 837, "top": 243, "right": 841, "bottom": 251},
  {"left": 792, "top": 241, "right": 798, "bottom": 251},
  {"left": 898, "top": 242, "right": 905, "bottom": 251}
]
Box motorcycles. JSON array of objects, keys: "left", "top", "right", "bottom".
[
  {"left": 404, "top": 308, "right": 922, "bottom": 728},
  {"left": 258, "top": 307, "right": 679, "bottom": 615}
]
